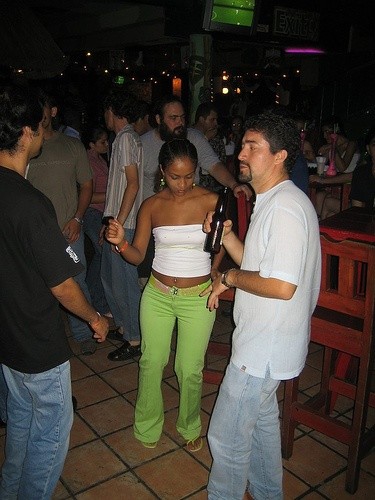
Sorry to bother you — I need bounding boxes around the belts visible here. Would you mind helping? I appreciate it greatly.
[150,273,211,296]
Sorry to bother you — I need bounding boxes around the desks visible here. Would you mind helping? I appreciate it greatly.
[309,173,353,212]
[319,205,374,414]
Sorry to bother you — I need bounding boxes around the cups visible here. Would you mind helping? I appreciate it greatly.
[316,151,327,174]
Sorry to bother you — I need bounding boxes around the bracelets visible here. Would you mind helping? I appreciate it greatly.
[72,216,80,222]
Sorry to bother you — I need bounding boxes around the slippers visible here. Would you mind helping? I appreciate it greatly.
[186,437,203,452]
[143,442,157,449]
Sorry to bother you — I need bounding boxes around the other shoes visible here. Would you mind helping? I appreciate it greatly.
[80,340,96,355]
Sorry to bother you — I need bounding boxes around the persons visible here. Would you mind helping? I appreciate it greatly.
[294,116,375,223]
[0,75,109,500]
[105,140,233,451]
[198,112,328,500]
[24,83,244,362]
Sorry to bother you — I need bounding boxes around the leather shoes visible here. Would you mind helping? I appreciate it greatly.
[107,327,129,343]
[108,342,140,362]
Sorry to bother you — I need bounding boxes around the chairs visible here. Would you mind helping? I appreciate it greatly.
[282,233,375,494]
[202,288,236,384]
[237,191,253,241]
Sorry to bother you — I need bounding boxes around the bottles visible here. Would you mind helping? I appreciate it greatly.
[204,186,230,254]
[327,133,338,177]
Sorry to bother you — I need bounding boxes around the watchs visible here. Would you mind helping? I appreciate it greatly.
[115,239,128,252]
[221,270,231,288]
